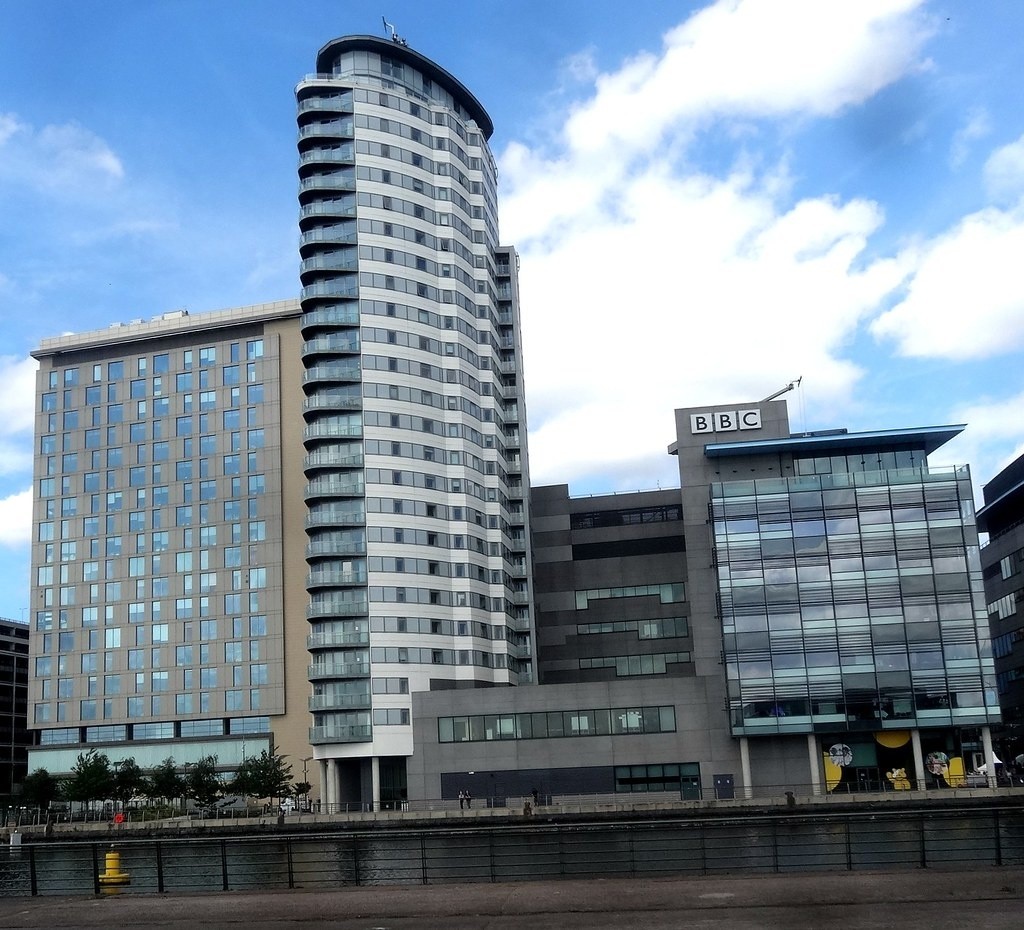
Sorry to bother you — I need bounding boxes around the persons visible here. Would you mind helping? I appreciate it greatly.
[316,796,321,812]
[459,791,464,809]
[530,785,540,807]
[464,790,472,809]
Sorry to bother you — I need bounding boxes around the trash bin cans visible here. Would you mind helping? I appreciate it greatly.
[680,776,702,800]
